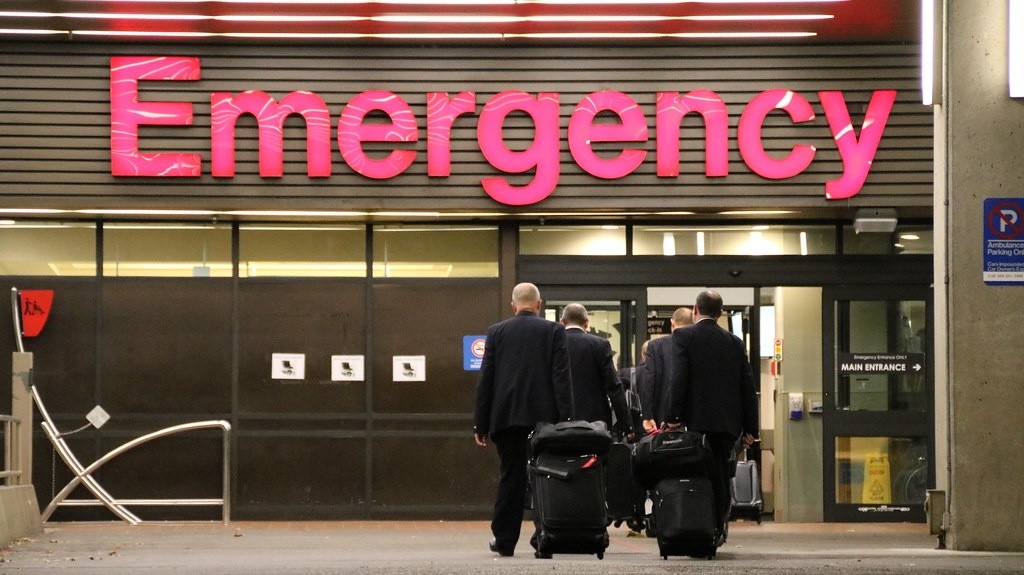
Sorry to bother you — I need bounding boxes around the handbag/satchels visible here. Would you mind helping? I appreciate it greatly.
[612,367,641,428]
[528,420,612,455]
[631,425,735,480]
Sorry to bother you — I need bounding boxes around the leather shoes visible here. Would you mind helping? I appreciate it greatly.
[489,540,514,557]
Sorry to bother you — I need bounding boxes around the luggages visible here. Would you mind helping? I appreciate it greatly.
[603,439,640,528]
[656,479,716,560]
[730,446,763,525]
[534,455,609,560]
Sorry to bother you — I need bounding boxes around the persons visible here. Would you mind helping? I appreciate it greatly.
[556,304,636,459]
[636,306,694,439]
[472,281,576,560]
[614,338,661,537]
[659,287,762,548]
